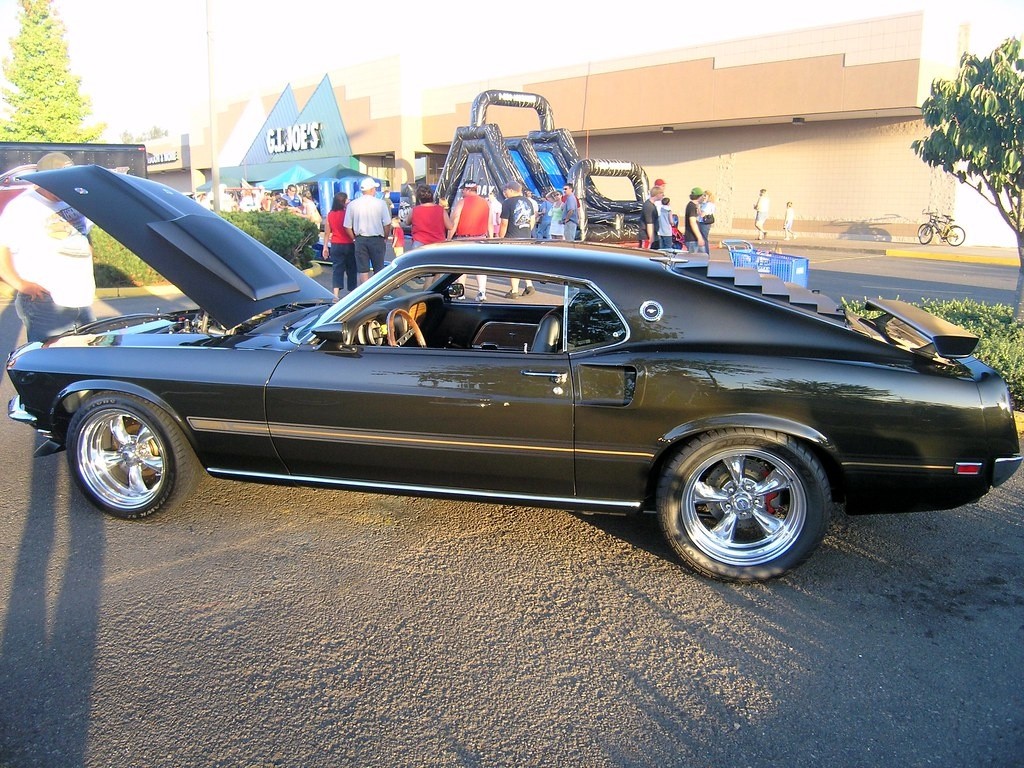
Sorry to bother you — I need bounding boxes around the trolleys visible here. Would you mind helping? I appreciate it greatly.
[722,240,809,289]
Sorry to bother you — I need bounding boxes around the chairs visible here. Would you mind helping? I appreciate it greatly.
[531,305,564,353]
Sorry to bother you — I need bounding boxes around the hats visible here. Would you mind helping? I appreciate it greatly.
[655,179,666,185]
[690,187,705,196]
[505,181,520,192]
[458,180,477,191]
[360,178,380,192]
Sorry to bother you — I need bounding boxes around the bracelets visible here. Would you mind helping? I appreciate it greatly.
[324,244,328,246]
[353,238,355,242]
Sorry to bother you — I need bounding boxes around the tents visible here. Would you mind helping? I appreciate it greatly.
[299,162,380,194]
[196,180,213,192]
[256,166,315,193]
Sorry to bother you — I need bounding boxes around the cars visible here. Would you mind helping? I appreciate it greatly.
[6,164,1023,585]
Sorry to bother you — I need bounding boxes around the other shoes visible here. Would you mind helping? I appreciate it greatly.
[784,237,789,240]
[763,232,768,240]
[755,235,762,240]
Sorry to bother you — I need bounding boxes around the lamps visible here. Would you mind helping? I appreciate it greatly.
[792,117,804,125]
[181,169,186,172]
[662,127,674,133]
[385,154,393,158]
[161,171,165,174]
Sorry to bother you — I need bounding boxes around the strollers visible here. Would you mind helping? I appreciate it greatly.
[672,226,689,251]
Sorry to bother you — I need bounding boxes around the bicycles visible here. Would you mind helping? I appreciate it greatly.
[918,209,966,246]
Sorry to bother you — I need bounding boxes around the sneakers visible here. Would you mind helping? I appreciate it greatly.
[521,286,536,296]
[452,295,467,300]
[504,290,520,300]
[475,290,487,302]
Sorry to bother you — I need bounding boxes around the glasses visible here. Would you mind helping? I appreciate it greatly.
[554,194,560,197]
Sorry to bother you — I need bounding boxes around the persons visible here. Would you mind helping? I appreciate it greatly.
[754,189,768,239]
[187,184,322,233]
[391,217,405,257]
[322,192,357,296]
[405,185,453,291]
[546,190,564,239]
[640,179,674,248]
[447,180,494,301]
[384,190,394,215]
[784,202,798,240]
[485,193,502,237]
[538,194,553,239]
[0,152,96,342]
[523,188,539,238]
[685,188,714,253]
[499,181,535,299]
[562,183,578,242]
[343,178,391,283]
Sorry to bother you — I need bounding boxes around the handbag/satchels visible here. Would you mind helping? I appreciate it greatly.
[547,208,554,217]
[703,214,715,224]
[535,215,543,223]
